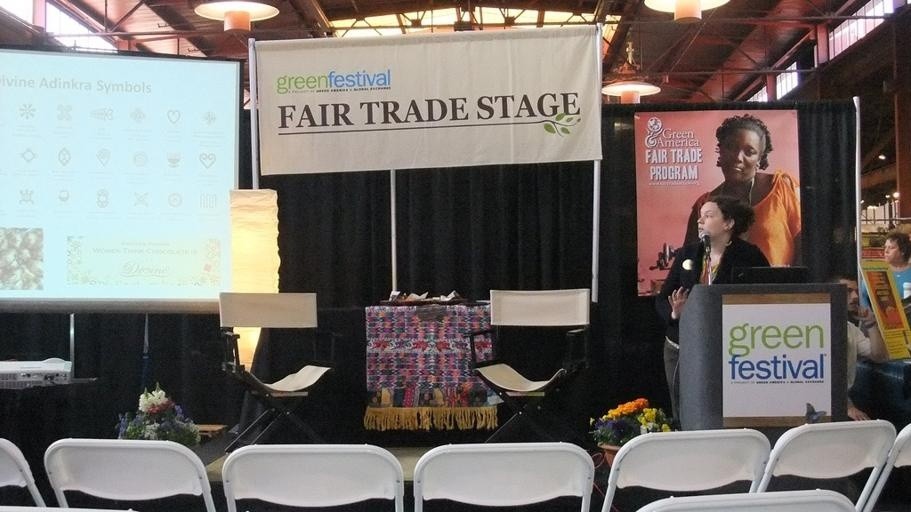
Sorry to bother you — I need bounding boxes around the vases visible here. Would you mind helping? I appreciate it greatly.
[597,442,622,468]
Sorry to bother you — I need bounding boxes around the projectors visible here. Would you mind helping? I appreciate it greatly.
[0,357,72,390]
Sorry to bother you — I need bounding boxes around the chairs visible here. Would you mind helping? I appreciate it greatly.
[222,444,405,512]
[43,438,216,512]
[1,436,46,507]
[635,489,855,512]
[863,423,911,511]
[757,420,897,512]
[413,442,595,512]
[602,429,771,512]
[462,288,590,443]
[220,331,331,452]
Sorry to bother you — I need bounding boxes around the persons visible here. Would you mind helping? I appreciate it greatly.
[823,271,890,421]
[861,233,911,317]
[656,195,770,424]
[682,115,802,267]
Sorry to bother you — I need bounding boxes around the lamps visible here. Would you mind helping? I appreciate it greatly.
[602,42,662,105]
[194,0,280,35]
[643,0,729,24]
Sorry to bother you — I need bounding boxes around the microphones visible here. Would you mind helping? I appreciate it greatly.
[699,231,713,256]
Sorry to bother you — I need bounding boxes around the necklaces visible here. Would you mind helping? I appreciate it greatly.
[717,178,754,207]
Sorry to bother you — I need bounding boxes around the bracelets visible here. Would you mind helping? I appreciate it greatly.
[863,319,876,328]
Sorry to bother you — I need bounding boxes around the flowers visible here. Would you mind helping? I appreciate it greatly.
[114,381,202,447]
[589,397,672,446]
